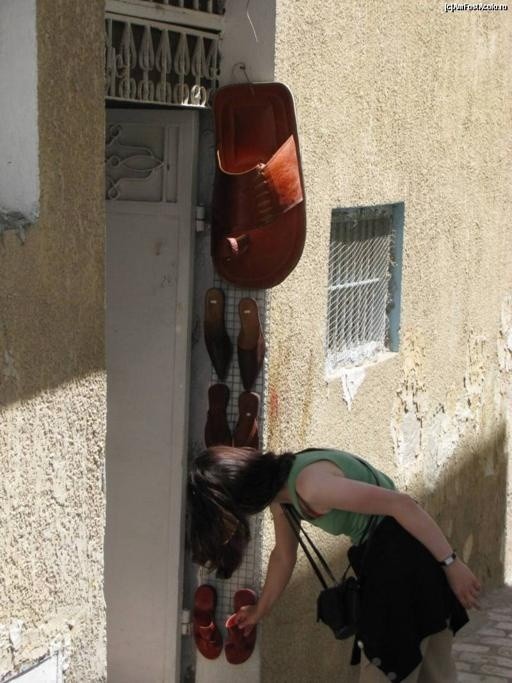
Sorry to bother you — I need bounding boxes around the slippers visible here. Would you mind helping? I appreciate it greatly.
[231,392,261,452]
[222,588,260,664]
[210,80,308,290]
[204,383,232,449]
[193,584,223,661]
[237,297,267,390]
[203,288,232,381]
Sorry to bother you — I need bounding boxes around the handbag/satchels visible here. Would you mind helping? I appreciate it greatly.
[315,573,366,640]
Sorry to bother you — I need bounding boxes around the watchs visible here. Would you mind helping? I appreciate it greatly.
[439,549,456,566]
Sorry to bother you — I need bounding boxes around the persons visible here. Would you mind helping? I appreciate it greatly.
[187,445,482,683]
